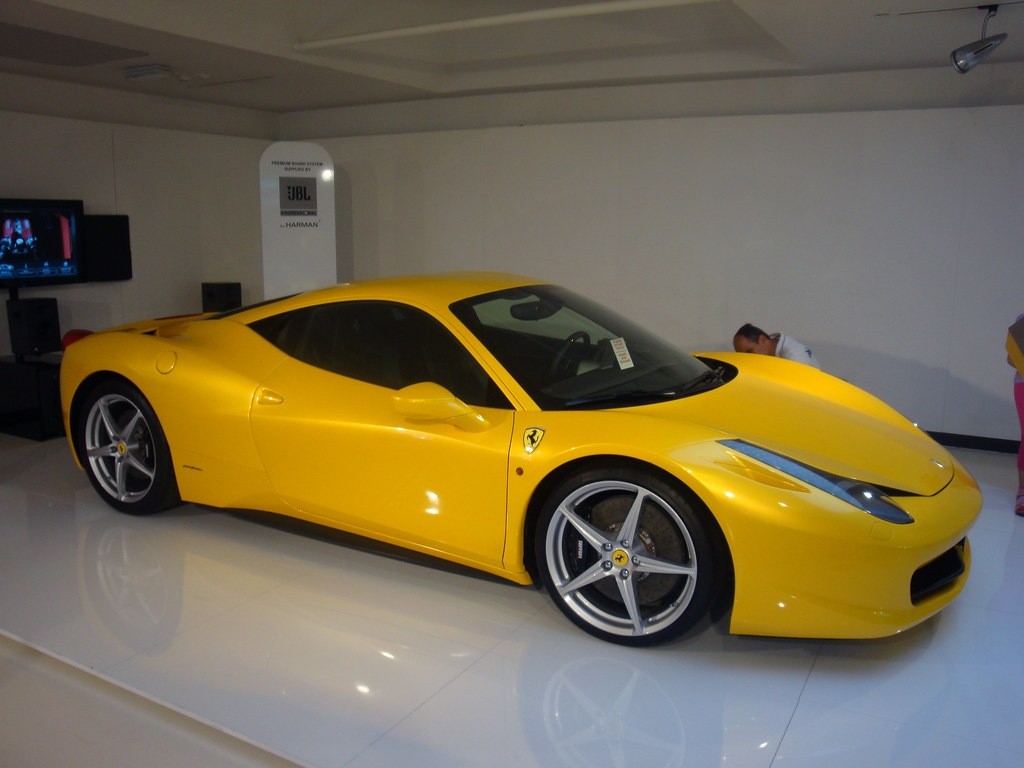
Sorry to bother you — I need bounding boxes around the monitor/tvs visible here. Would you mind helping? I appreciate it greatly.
[70,215,133,284]
[0,197,86,287]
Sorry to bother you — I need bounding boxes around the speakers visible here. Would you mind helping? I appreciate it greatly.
[201,281,242,313]
[7,298,62,362]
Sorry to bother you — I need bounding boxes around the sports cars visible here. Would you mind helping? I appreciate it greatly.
[58,271,987,649]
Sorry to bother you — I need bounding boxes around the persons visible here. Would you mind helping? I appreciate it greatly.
[733,323,820,370]
[1006,314,1024,515]
